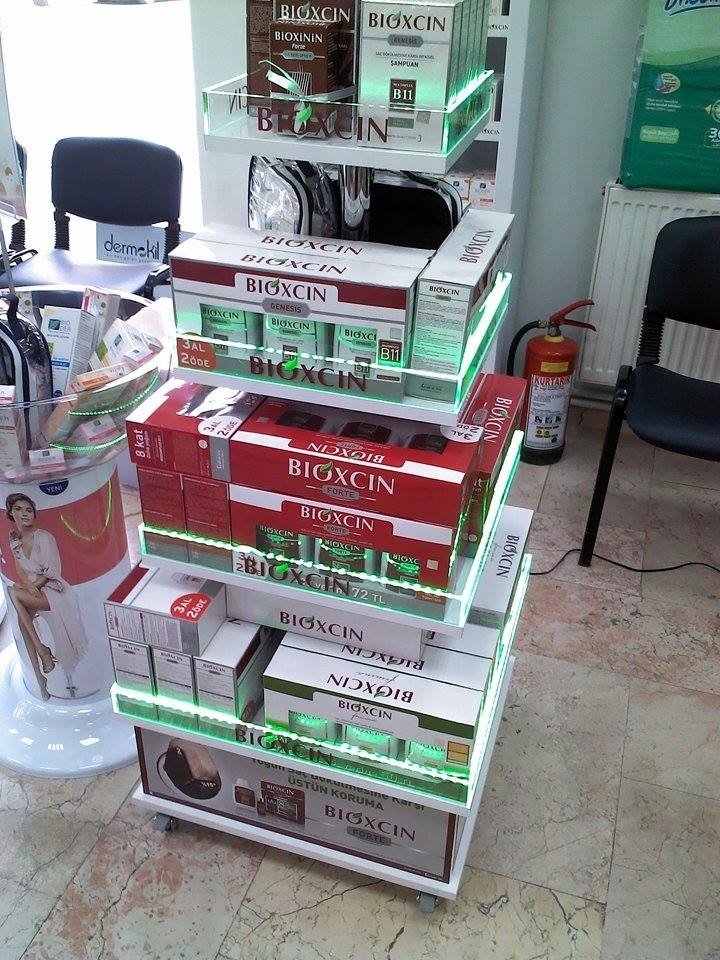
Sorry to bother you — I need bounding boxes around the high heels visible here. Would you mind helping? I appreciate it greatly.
[38,676,52,702]
[37,645,58,674]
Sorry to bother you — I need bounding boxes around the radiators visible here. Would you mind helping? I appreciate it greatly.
[575,179,720,398]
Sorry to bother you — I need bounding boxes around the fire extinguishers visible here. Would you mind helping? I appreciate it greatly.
[506,300,596,466]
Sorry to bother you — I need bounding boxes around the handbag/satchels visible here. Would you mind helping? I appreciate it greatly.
[326,158,462,249]
[245,153,338,240]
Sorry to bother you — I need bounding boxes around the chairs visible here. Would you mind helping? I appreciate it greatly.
[0,135,185,338]
[576,215,720,579]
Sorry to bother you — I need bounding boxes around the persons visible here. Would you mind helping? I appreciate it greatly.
[3,493,89,703]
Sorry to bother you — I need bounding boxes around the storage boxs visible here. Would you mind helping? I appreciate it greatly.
[0,284,167,485]
[103,0,538,888]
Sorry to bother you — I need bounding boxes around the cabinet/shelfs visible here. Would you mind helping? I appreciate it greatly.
[85,2,551,913]
